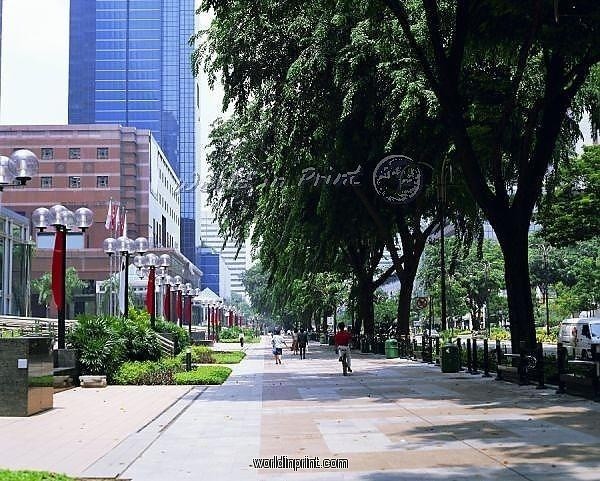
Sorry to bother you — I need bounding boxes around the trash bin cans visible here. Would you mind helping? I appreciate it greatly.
[320,335,326,344]
[329,334,335,346]
[384,338,399,359]
[440,344,460,373]
[360,338,369,353]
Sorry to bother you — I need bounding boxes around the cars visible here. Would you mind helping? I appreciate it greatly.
[423,329,439,341]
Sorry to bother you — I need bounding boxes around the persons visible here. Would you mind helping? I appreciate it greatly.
[571,327,579,347]
[335,321,353,372]
[238,330,245,347]
[259,326,351,364]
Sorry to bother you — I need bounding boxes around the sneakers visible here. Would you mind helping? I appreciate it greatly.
[348,367,352,373]
[276,359,281,364]
[339,356,342,362]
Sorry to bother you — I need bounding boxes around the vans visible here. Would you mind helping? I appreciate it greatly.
[556,317,600,362]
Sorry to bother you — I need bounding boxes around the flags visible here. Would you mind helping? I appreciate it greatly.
[104,200,127,239]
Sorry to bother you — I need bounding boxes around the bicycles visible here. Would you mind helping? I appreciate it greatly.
[339,349,351,376]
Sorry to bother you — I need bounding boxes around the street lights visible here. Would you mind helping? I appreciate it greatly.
[31,203,94,350]
[103,234,236,345]
